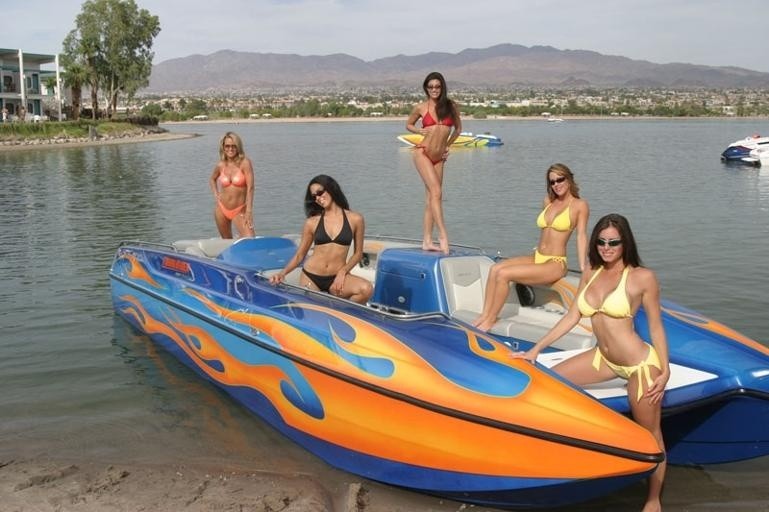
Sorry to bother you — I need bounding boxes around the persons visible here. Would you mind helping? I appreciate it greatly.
[1,107,10,123]
[470,164,589,333]
[19,105,26,122]
[507,212,673,512]
[404,70,462,256]
[267,174,375,306]
[208,131,257,239]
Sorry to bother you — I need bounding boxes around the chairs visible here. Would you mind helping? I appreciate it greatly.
[442,253,595,350]
[258,267,303,288]
[172,240,233,259]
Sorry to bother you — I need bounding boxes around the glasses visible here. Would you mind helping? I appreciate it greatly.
[427,85,441,89]
[550,176,565,185]
[597,237,622,246]
[311,188,326,201]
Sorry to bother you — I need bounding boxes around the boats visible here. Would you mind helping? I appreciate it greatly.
[109,236,769,511]
[396,131,504,149]
[547,118,564,123]
[721,135,769,162]
[740,144,769,165]
